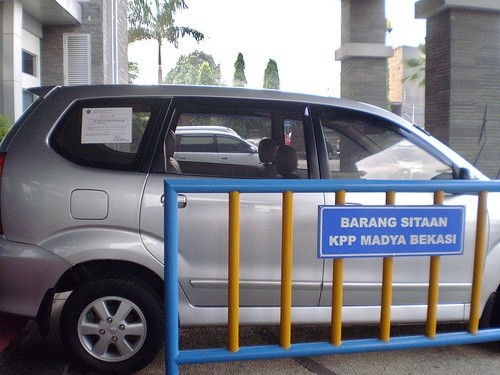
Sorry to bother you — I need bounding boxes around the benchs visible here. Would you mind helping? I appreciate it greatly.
[168,129,189,174]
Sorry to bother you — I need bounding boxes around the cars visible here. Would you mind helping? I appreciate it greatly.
[0,84,499,375]
[166,129,267,167]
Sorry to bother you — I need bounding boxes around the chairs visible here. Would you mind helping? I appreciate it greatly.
[256,138,277,179]
[275,145,307,181]
